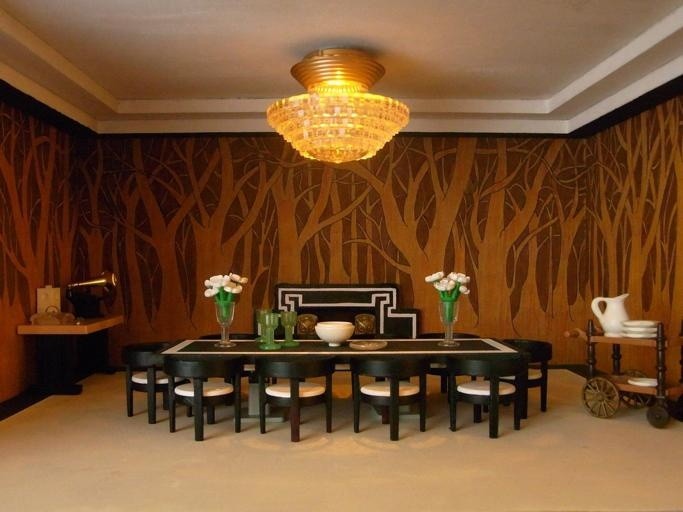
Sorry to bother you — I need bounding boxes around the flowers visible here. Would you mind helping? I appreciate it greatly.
[424,270,471,322]
[204,272,248,321]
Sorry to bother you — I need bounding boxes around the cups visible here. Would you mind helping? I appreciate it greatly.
[257,313,280,349]
[255,309,269,342]
[279,312,299,347]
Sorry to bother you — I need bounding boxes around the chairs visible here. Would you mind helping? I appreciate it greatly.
[199,333,258,406]
[351,356,427,441]
[418,333,481,393]
[122,343,191,424]
[447,356,521,438]
[499,339,552,419]
[164,355,241,441]
[272,334,305,385]
[255,357,335,442]
[350,334,388,381]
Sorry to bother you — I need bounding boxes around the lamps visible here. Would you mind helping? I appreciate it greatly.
[266,48,410,164]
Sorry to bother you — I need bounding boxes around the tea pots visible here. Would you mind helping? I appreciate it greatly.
[591,294,629,338]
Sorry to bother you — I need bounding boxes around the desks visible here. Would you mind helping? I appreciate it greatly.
[16,312,125,395]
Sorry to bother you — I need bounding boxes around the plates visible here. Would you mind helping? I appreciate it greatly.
[628,378,657,387]
[349,341,387,350]
[622,320,659,340]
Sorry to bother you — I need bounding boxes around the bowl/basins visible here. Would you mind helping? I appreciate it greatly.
[314,321,354,347]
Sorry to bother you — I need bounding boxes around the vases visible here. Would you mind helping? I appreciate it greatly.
[214,301,237,348]
[437,301,460,347]
